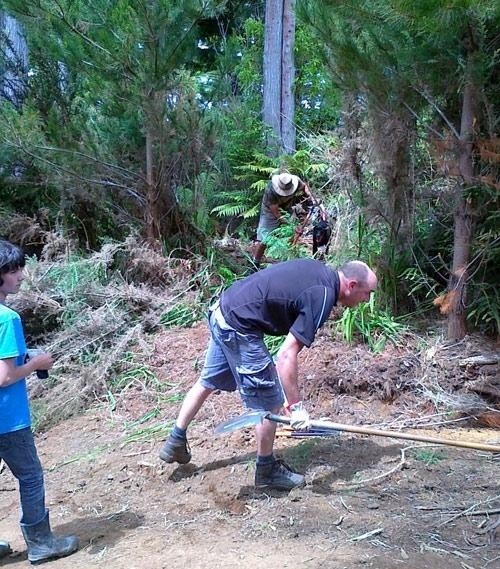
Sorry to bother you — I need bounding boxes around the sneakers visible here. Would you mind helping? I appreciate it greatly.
[159,439,192,465]
[255,460,304,491]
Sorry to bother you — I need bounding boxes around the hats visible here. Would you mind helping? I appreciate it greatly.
[271,171,299,196]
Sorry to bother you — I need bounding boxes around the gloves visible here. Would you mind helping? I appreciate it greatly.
[288,401,311,432]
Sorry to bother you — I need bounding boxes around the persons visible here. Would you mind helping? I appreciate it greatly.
[1,239,82,566]
[252,170,316,270]
[156,258,379,489]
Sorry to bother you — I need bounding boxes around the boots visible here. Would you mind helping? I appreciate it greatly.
[0,540,11,558]
[18,509,80,563]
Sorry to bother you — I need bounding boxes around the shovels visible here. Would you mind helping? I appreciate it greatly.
[214,411,500,452]
